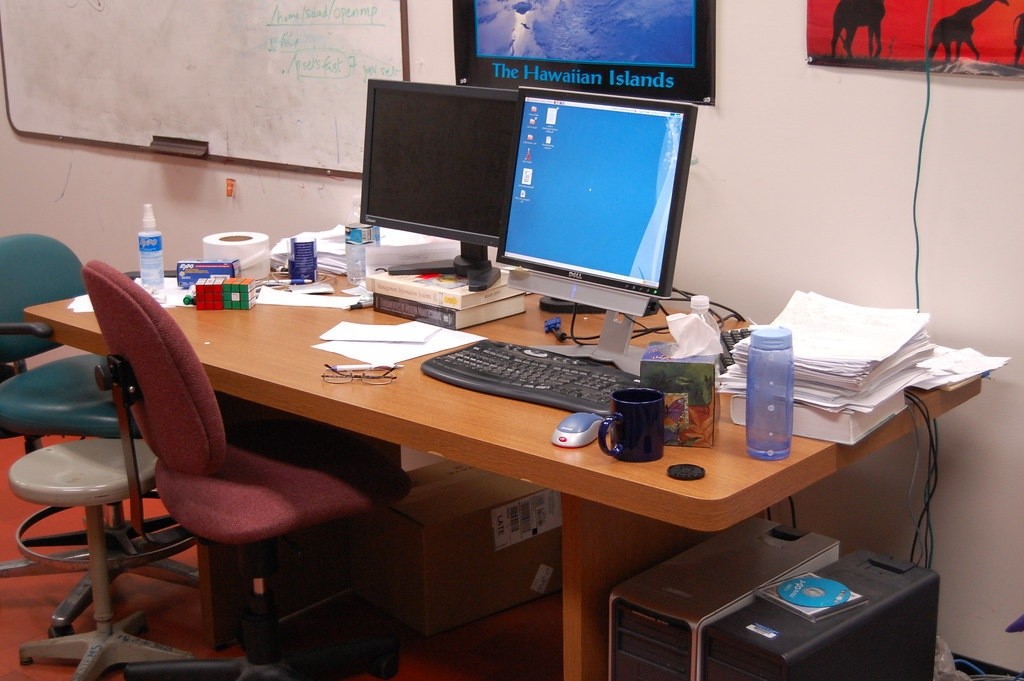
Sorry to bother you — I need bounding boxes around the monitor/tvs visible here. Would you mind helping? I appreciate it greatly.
[492,85,699,377]
[358,78,518,291]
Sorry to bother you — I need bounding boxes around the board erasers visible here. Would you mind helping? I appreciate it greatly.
[149,135,209,157]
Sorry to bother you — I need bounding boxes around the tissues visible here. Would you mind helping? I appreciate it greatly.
[639,311,725,449]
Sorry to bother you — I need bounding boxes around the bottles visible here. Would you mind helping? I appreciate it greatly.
[138,204,165,289]
[747,327,794,462]
[344,195,381,287]
[689,294,722,425]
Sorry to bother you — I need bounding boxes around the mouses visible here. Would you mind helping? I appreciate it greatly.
[551,412,609,448]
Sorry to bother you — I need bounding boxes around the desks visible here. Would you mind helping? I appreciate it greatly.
[23,253,982,681]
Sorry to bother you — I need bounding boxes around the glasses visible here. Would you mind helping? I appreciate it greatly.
[321,364,399,384]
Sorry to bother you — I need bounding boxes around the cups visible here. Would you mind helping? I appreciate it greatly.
[598,387,665,462]
[286,236,318,282]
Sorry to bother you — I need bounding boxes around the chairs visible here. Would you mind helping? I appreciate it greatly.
[1,233,150,449]
[79,260,401,681]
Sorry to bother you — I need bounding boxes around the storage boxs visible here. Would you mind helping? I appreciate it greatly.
[370,457,563,635]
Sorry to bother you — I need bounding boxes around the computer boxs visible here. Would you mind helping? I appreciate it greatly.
[608,516,839,681]
[703,548,940,681]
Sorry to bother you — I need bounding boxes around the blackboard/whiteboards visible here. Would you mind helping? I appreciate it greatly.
[0,0,410,178]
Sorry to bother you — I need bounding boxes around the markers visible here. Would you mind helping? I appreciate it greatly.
[183,285,196,305]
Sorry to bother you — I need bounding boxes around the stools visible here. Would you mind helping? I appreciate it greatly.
[9,439,196,681]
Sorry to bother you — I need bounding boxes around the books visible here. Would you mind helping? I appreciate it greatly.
[730,386,908,445]
[365,272,527,331]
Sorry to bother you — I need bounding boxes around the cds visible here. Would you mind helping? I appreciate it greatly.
[777,577,851,607]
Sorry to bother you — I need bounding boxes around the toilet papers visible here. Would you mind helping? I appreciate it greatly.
[203,230,272,280]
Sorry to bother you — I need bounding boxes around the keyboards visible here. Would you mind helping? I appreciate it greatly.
[420,339,641,418]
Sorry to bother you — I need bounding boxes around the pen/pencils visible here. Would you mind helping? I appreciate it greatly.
[327,364,405,371]
[350,301,373,310]
[263,279,313,285]
